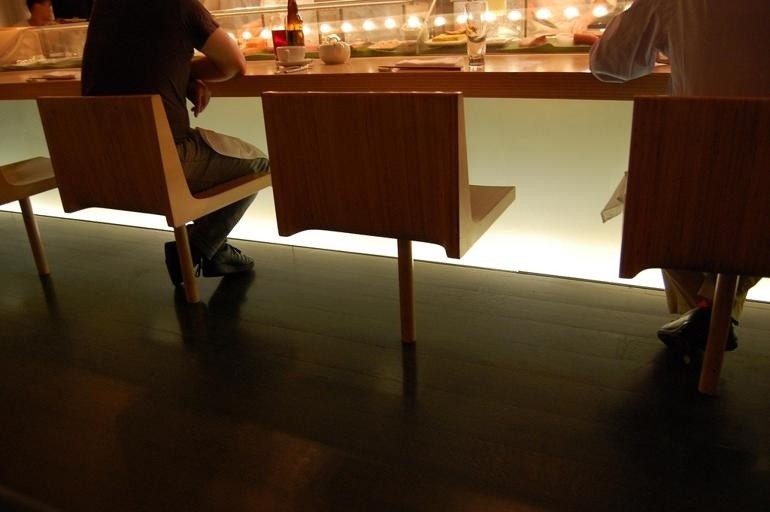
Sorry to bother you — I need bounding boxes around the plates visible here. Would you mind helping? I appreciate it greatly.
[368,40,402,51]
[277,58,314,68]
[378,55,463,70]
[424,30,592,49]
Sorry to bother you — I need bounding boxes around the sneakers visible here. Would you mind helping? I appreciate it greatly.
[202,244,255,277]
[164,241,185,287]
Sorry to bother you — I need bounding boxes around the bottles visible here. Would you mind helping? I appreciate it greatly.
[286,0,304,46]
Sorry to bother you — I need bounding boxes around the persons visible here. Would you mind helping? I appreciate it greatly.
[80,0,274,293]
[583,1,768,353]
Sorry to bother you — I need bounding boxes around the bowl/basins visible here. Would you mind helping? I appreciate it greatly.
[319,44,351,64]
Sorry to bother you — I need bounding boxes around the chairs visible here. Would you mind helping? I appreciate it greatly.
[618,94,770,396]
[36,92,272,328]
[260,90,516,347]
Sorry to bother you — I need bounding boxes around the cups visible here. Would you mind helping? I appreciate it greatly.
[276,45,307,63]
[463,1,486,67]
[270,16,288,56]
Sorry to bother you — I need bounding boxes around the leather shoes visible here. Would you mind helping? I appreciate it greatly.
[724,325,737,352]
[657,307,710,349]
